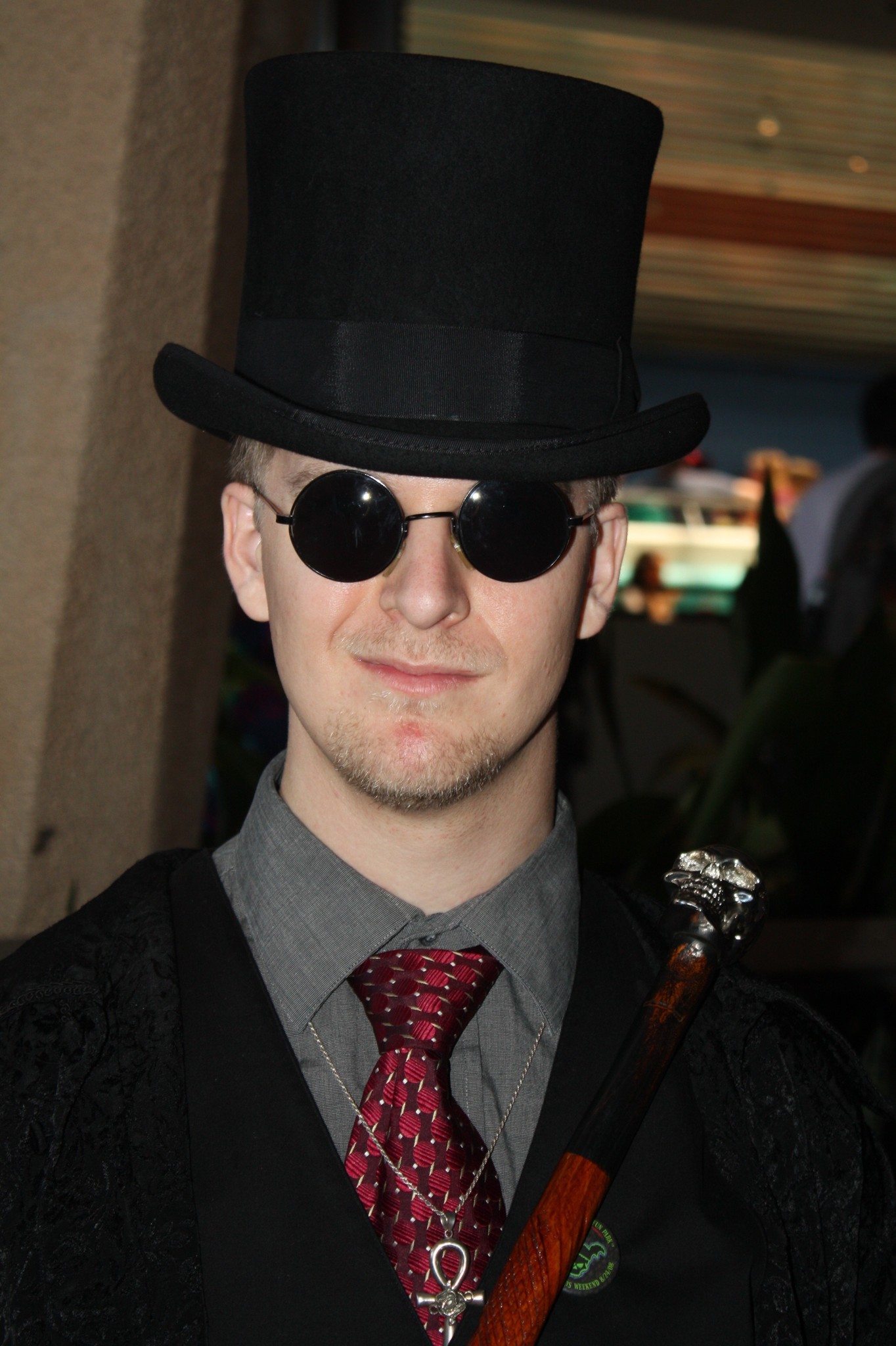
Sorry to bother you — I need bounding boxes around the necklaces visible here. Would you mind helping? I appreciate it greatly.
[307,1022,545,1346]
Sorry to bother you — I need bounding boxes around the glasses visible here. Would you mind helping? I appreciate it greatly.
[241,472,617,584]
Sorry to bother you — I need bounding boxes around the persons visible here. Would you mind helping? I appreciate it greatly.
[0,47,896,1346]
[786,363,896,611]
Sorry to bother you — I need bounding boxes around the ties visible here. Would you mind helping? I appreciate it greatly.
[341,943,511,1346]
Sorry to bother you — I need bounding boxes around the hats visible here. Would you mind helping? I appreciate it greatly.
[156,43,716,482]
